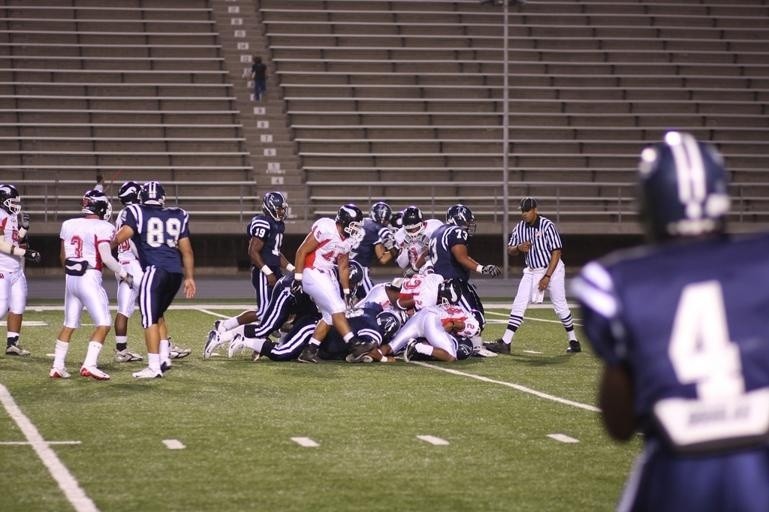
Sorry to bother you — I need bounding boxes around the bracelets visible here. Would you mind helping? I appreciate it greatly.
[545,274,551,277]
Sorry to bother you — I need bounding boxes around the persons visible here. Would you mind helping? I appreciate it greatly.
[201,189,504,365]
[113,180,192,363]
[484,198,583,354]
[49,190,134,380]
[0,182,42,356]
[94,176,110,193]
[110,180,197,379]
[570,130,769,511]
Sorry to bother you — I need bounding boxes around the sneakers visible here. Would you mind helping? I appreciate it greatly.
[403,337,420,366]
[111,348,146,363]
[47,366,74,380]
[78,362,112,381]
[202,330,220,362]
[564,338,583,355]
[3,343,32,359]
[344,338,397,364]
[166,335,194,361]
[215,320,228,350]
[228,332,246,360]
[250,334,272,363]
[484,338,513,357]
[297,345,327,365]
[131,353,173,381]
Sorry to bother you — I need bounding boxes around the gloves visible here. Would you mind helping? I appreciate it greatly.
[402,264,420,278]
[344,289,353,309]
[22,248,42,265]
[401,234,413,250]
[476,262,503,281]
[417,232,431,248]
[123,271,134,291]
[21,212,32,230]
[290,277,304,297]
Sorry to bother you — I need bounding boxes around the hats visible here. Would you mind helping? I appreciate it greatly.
[516,195,538,212]
[96,174,103,183]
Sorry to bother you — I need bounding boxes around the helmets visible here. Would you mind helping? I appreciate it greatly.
[140,180,167,206]
[468,307,486,332]
[261,189,289,224]
[635,128,735,239]
[0,183,22,215]
[445,203,476,227]
[335,202,366,237]
[438,277,463,304]
[455,336,474,360]
[370,201,425,231]
[347,261,364,286]
[375,311,400,343]
[117,180,142,199]
[80,189,113,222]
